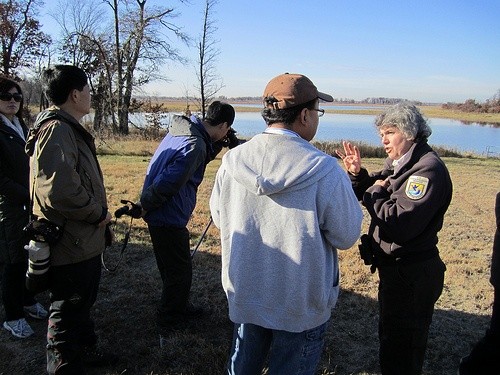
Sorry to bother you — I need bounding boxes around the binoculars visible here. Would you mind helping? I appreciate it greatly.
[114,205,142,219]
[358,234,379,266]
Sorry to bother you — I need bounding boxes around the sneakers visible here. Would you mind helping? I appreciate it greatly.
[23,301,48,320]
[3,318,34,338]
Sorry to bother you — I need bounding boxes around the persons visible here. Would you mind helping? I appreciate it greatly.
[91,86,105,131]
[0,77,48,338]
[209,73,363,375]
[459,191,500,375]
[140,101,235,326]
[334,103,453,375]
[226,126,239,148]
[27,66,119,375]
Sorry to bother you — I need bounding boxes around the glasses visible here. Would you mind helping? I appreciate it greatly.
[0,92,23,103]
[310,107,325,118]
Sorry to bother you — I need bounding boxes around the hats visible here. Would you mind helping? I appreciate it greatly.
[264,73,334,110]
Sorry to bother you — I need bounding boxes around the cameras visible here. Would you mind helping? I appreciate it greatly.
[23,218,62,294]
[217,130,248,150]
